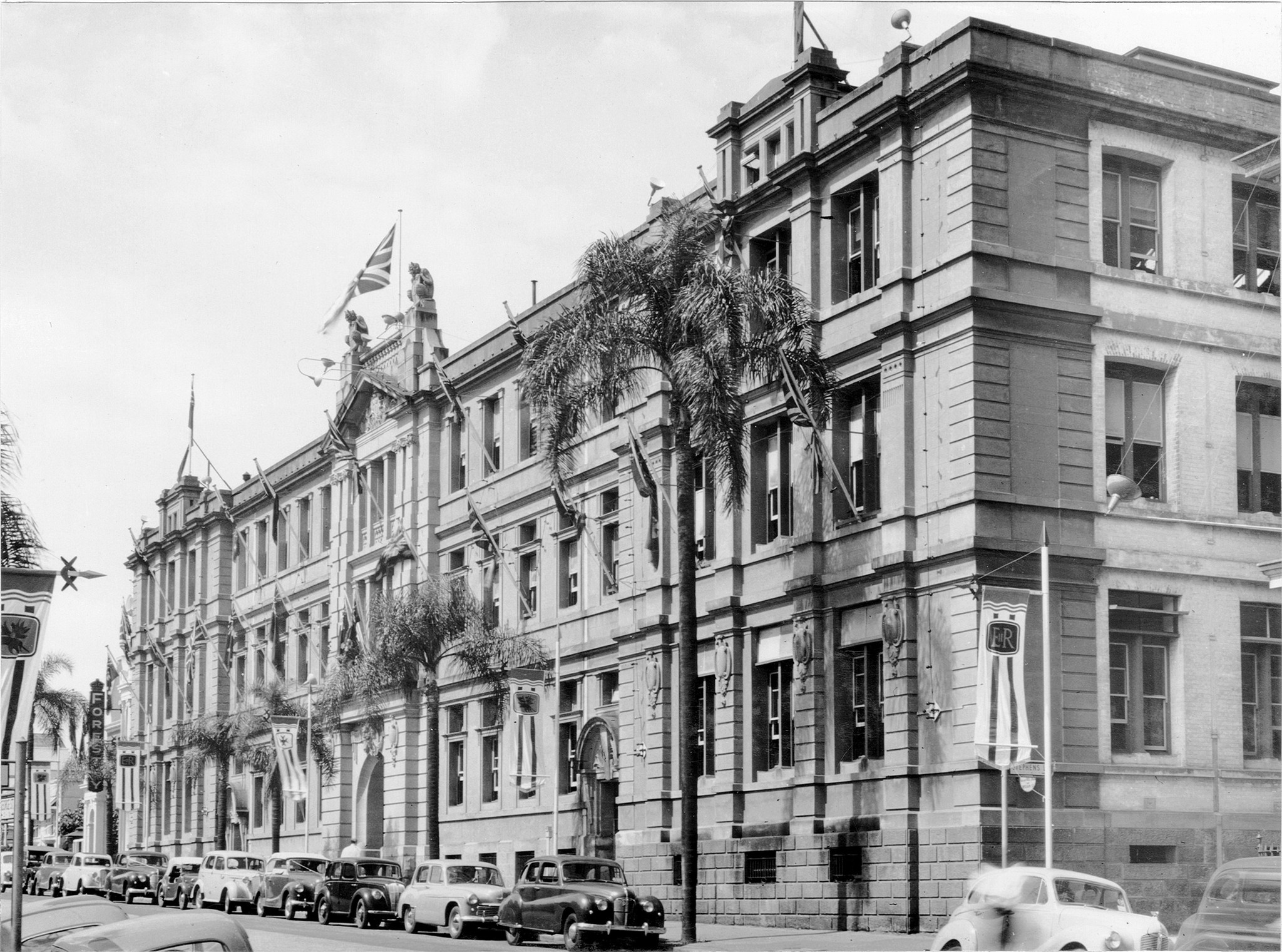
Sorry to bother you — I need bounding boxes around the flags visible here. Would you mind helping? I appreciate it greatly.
[434,357,461,424]
[216,492,240,563]
[345,591,362,665]
[551,468,586,540]
[627,424,657,497]
[505,306,530,351]
[118,601,136,672]
[703,179,736,255]
[185,613,209,688]
[222,599,248,677]
[317,222,396,337]
[373,523,418,585]
[268,582,290,684]
[337,599,350,665]
[106,652,120,716]
[256,462,280,545]
[466,498,497,554]
[327,416,362,503]
[777,349,816,427]
[146,630,171,700]
[132,533,154,579]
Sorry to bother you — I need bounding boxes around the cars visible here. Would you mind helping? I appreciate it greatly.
[50,908,253,952]
[492,855,667,952]
[251,852,334,920]
[928,862,1172,951]
[190,850,268,914]
[99,849,170,905]
[1169,855,1281,951]
[0,844,115,898]
[154,856,214,910]
[0,892,130,952]
[312,857,409,929]
[394,858,513,940]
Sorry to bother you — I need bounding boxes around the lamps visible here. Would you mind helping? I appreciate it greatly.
[1106,473,1142,515]
